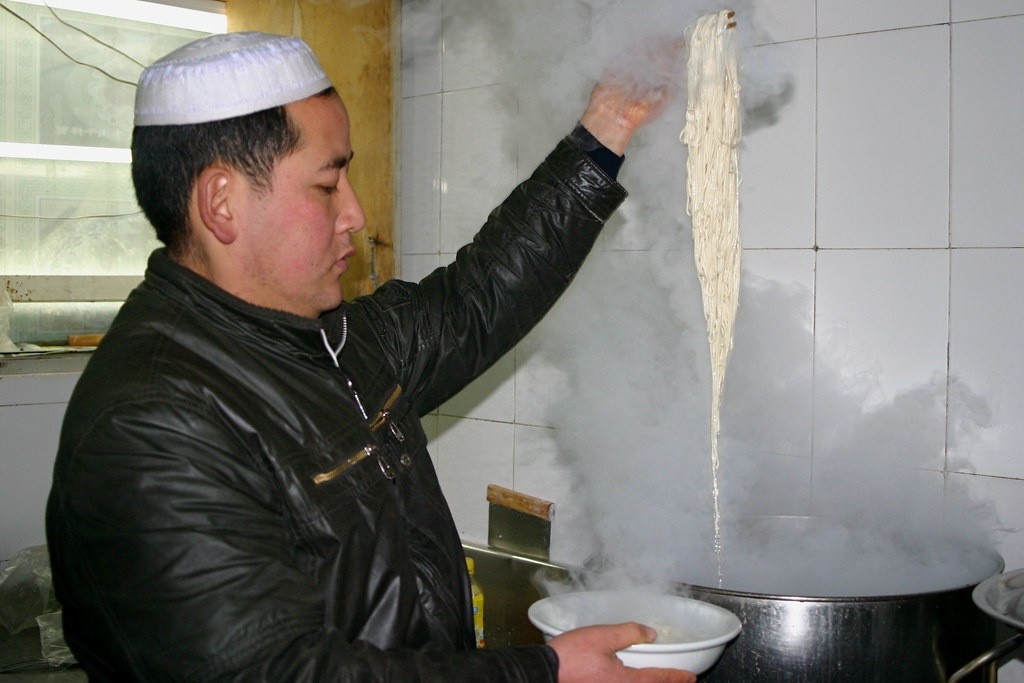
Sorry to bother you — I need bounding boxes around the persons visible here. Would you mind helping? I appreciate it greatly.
[42,29,700,683]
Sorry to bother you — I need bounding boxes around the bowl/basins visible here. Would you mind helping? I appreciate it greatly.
[526,590,743,678]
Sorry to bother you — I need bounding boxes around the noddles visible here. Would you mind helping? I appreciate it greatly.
[678,9,746,544]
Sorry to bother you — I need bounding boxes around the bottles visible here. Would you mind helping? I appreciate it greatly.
[465,557,484,650]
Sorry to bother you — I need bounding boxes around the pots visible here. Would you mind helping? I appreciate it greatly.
[657,538,1005,683]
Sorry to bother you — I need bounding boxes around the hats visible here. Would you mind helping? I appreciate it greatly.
[130,29,335,133]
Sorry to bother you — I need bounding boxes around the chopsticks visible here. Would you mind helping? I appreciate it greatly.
[663,10,738,50]
